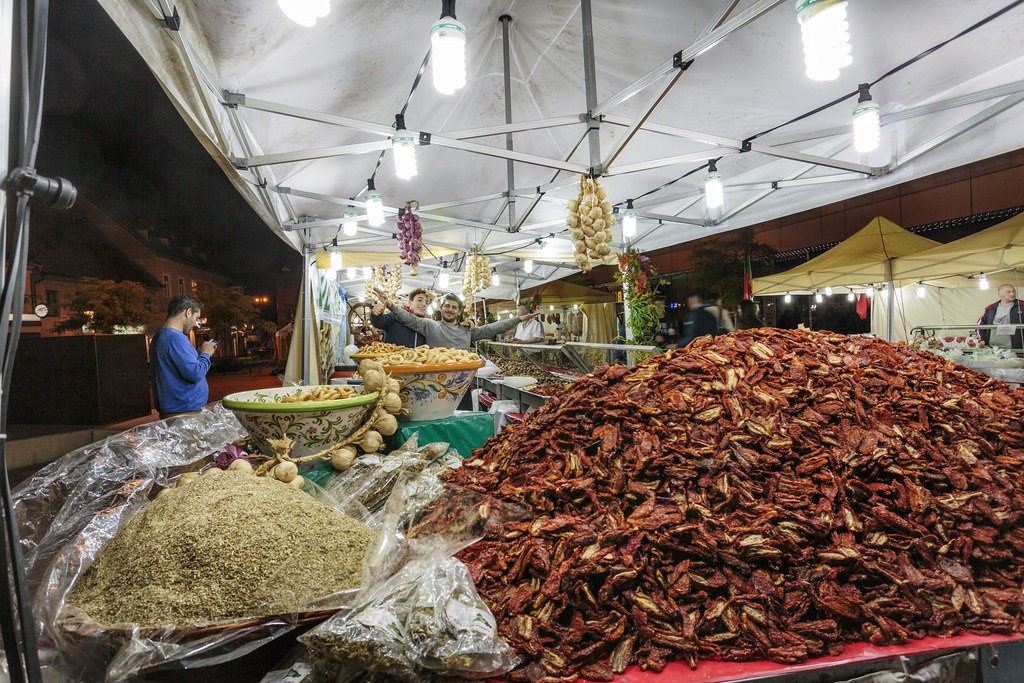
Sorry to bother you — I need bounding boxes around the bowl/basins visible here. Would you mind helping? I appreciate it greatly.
[384,360,485,421]
[223,384,381,462]
[350,354,395,365]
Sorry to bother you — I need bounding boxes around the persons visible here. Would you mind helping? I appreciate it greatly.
[654,296,680,353]
[510,305,546,355]
[666,292,717,350]
[975,283,1024,348]
[369,287,436,347]
[703,286,736,335]
[734,299,767,330]
[370,287,541,413]
[150,294,217,419]
[614,325,628,365]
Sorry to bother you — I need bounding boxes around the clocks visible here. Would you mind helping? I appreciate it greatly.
[34,303,48,318]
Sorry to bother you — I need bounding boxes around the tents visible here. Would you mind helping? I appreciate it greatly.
[884,209,1024,344]
[472,279,617,367]
[749,214,944,341]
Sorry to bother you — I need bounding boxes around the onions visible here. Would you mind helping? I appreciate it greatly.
[394,208,422,277]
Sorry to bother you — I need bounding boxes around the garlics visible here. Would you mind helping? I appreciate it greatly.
[566,187,614,273]
[362,265,400,302]
[462,257,490,313]
[157,359,400,503]
